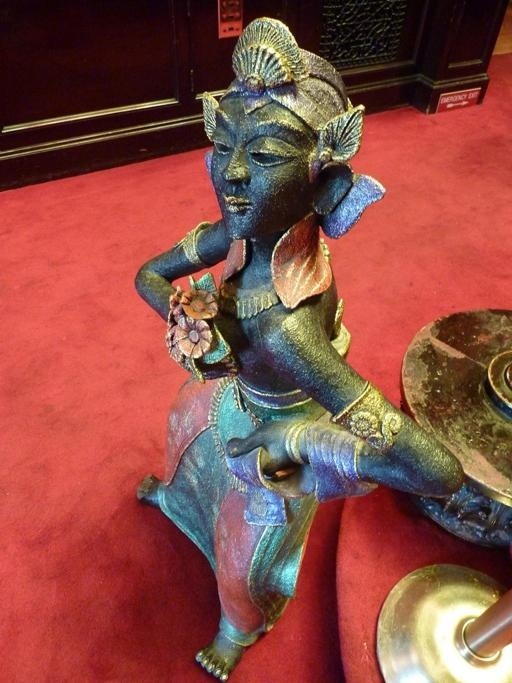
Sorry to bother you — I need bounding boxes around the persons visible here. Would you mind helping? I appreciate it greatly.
[133,16,465,683]
